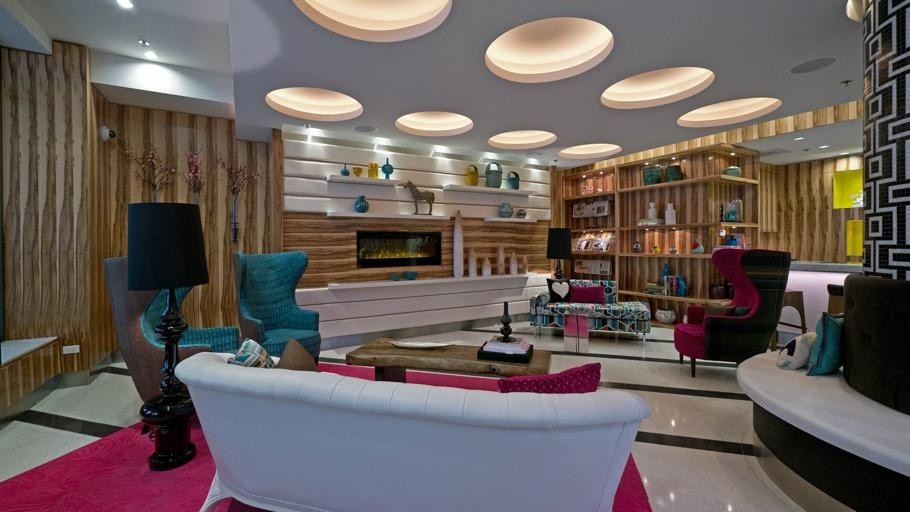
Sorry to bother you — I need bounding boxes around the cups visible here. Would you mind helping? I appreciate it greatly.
[668,275,678,296]
[368,163,379,179]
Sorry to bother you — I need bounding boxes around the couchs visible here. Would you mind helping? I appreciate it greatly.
[172,351,650,512]
[527,279,652,343]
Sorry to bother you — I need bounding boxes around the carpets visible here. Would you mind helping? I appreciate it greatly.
[2,361,655,512]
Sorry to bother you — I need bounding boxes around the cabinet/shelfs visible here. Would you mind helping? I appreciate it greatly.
[560,143,760,308]
[326,173,541,224]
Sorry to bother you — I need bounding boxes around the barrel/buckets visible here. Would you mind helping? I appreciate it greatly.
[664,165,682,182]
[643,164,662,185]
[464,165,478,186]
[506,171,520,190]
[485,161,503,187]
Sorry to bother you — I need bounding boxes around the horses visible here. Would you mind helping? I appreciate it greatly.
[403,180,436,214]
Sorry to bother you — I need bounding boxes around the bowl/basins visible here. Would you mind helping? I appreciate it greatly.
[353,167,363,178]
[391,274,400,281]
[405,271,416,279]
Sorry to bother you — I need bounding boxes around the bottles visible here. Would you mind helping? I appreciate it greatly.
[468,245,477,277]
[521,254,529,274]
[648,201,657,220]
[495,245,506,275]
[664,203,676,225]
[452,210,464,277]
[481,256,492,276]
[663,262,669,295]
[731,198,743,222]
[508,251,518,275]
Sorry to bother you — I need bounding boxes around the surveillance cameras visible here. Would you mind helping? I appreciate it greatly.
[101,128,116,142]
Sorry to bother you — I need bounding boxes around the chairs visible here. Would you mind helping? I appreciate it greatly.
[673,247,791,379]
[104,256,241,403]
[233,251,322,370]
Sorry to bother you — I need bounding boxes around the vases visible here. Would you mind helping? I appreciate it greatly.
[195,193,201,208]
[231,196,238,243]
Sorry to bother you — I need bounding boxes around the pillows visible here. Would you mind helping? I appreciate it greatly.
[735,306,753,317]
[805,311,846,377]
[226,337,276,369]
[545,277,571,303]
[774,332,816,370]
[496,361,601,393]
[570,285,606,304]
[702,299,737,316]
[276,337,319,370]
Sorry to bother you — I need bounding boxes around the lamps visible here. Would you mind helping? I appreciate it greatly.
[547,227,575,278]
[126,201,210,471]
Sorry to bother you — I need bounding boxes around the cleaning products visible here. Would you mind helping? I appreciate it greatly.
[676,274,686,296]
[648,202,676,225]
[659,264,671,285]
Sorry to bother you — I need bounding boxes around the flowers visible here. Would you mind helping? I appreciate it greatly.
[184,149,204,191]
[114,133,173,200]
[214,155,268,196]
